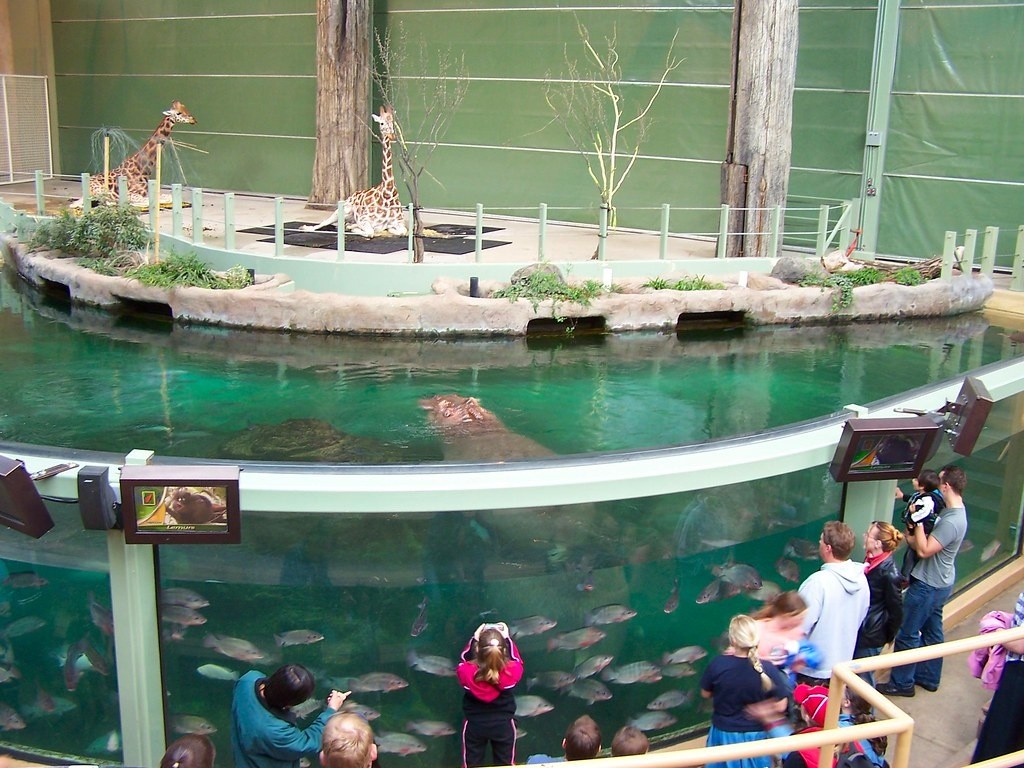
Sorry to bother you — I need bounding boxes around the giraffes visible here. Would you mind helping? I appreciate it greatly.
[297,106,407,239]
[89,101,197,201]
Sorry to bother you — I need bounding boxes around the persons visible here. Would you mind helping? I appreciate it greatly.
[319,710,377,768]
[796,518,869,689]
[230,664,352,768]
[159,733,216,768]
[853,521,904,715]
[972,592,1024,768]
[456,621,524,768]
[700,591,888,768]
[877,465,967,695]
[611,726,649,757]
[526,715,601,764]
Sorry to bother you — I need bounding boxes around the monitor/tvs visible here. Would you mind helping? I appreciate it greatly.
[829,416,940,483]
[0,455,54,539]
[946,376,995,456]
[118,465,241,545]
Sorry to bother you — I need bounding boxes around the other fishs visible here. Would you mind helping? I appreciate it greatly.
[0,537,1024,758]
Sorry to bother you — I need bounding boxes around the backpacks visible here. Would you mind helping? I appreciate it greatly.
[834,742,877,768]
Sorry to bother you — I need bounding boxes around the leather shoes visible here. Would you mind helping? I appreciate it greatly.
[875,683,915,697]
[914,682,937,692]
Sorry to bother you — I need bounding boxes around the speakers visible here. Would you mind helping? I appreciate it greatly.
[922,414,944,463]
[77,467,117,530]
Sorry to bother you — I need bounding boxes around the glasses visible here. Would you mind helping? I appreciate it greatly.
[863,533,877,540]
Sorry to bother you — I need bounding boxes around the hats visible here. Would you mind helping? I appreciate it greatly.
[794,684,829,727]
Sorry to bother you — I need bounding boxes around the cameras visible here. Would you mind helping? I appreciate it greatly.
[482,624,504,632]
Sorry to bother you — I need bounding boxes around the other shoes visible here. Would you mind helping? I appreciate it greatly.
[901,581,909,590]
